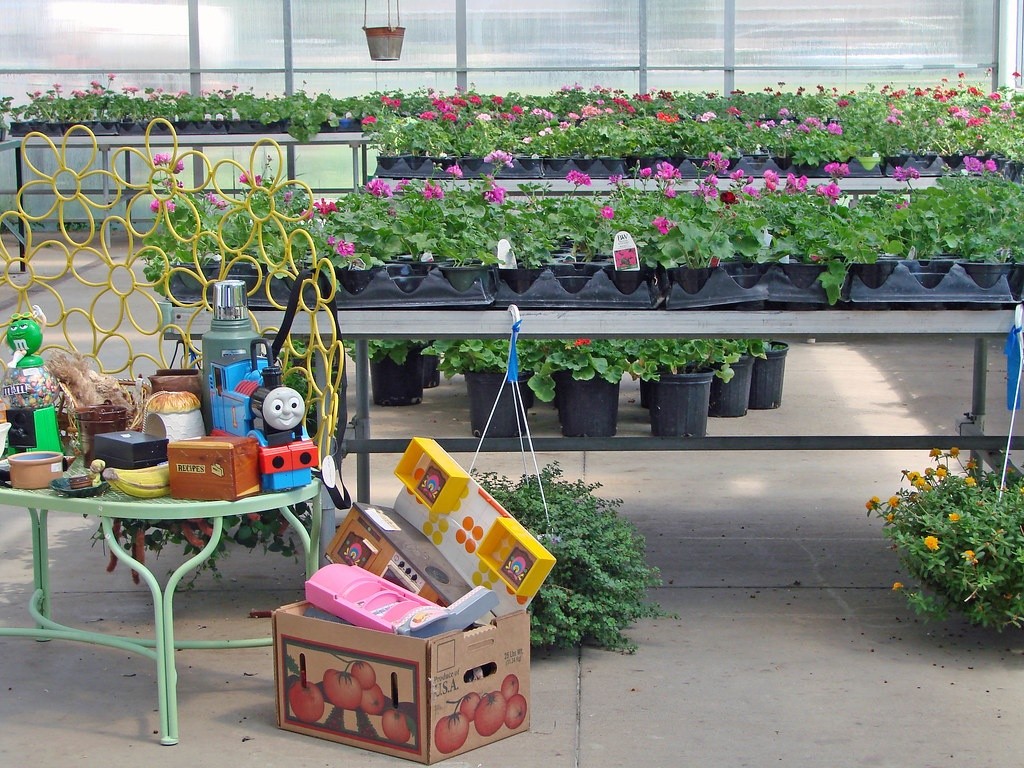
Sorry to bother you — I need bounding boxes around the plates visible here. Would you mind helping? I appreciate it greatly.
[49,477,109,497]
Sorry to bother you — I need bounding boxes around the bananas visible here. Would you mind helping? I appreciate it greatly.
[102,464,171,499]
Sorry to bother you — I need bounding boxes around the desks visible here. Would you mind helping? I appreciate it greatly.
[372,178,1021,193]
[0,479,323,743]
[157,305,1024,505]
[0,138,26,272]
[11,133,378,235]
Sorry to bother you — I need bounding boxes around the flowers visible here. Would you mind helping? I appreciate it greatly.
[0,73,1024,255]
[866,447,1024,634]
[573,339,590,348]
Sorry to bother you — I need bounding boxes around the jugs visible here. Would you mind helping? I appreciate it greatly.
[203,280,262,435]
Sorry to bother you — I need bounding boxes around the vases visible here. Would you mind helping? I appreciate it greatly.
[160,262,1021,310]
[727,158,739,170]
[572,158,591,172]
[379,156,401,170]
[970,155,985,164]
[601,158,626,172]
[744,154,769,169]
[518,158,542,171]
[900,154,919,169]
[410,155,430,170]
[991,155,1005,167]
[688,158,709,169]
[997,158,1010,171]
[947,155,963,168]
[460,157,484,172]
[365,27,404,61]
[928,156,946,168]
[1004,162,1022,180]
[368,343,790,440]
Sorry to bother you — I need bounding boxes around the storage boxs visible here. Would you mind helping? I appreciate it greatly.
[271,600,530,765]
[168,436,260,504]
[96,430,169,481]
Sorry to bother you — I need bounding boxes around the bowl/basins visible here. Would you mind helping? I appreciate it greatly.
[8,451,64,489]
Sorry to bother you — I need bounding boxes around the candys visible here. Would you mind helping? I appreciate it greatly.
[0,364,60,410]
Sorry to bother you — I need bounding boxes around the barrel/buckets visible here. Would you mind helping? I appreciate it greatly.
[74,400,134,468]
[366,27,406,60]
